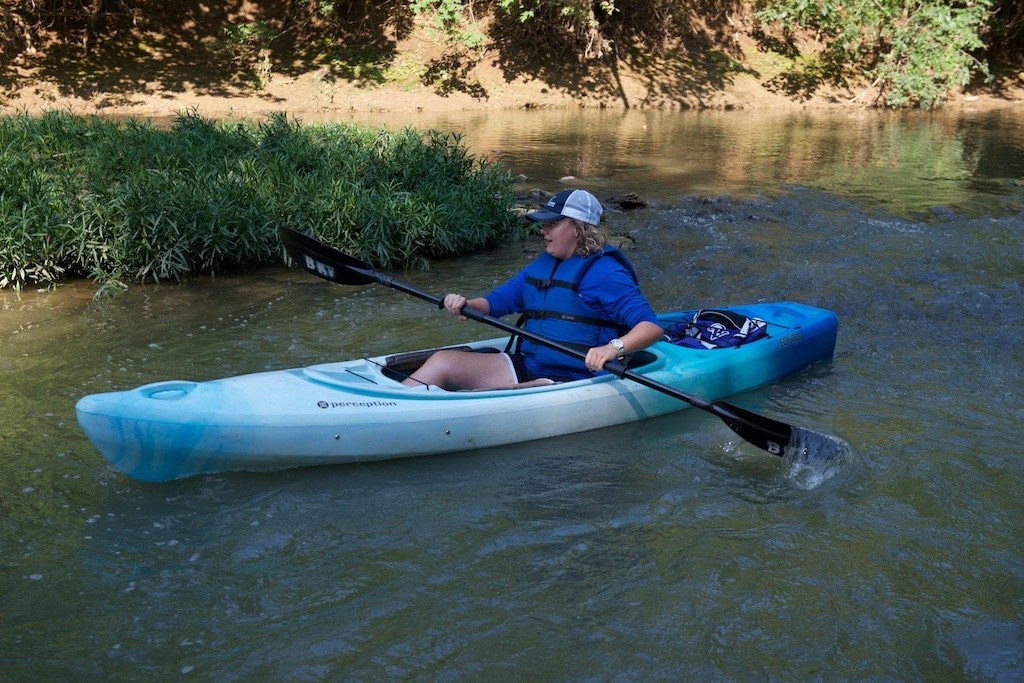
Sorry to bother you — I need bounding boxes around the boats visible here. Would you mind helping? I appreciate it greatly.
[76,299,840,480]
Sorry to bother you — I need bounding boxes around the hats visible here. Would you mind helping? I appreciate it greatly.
[525,188,604,227]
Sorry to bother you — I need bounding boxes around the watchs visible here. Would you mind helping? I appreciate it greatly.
[610,339,624,360]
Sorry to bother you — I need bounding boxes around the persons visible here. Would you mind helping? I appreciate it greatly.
[399,188,664,386]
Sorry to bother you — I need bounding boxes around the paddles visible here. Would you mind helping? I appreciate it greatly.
[278,228,791,456]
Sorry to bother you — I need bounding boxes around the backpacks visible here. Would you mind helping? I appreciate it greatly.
[658,307,768,350]
